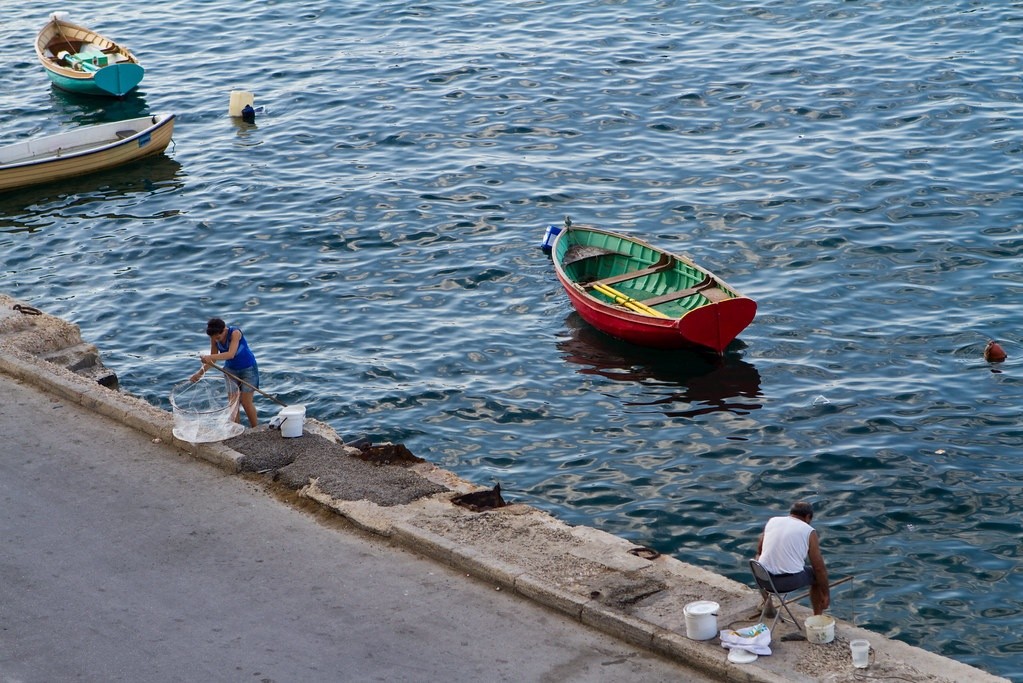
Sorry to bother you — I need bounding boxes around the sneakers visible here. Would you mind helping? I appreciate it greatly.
[757,601,776,618]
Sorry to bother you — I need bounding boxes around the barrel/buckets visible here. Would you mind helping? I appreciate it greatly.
[277,411,303,438]
[681,601,723,643]
[804,615,837,645]
[282,403,307,422]
[539,225,561,250]
[230,89,255,119]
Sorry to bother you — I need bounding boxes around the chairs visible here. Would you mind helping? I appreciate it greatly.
[749,559,802,635]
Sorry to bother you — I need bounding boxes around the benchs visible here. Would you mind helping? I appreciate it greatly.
[117,130,138,138]
[615,275,715,310]
[48,44,117,60]
[581,253,673,288]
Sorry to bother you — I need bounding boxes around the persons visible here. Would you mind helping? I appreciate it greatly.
[751,500,832,622]
[186,316,261,431]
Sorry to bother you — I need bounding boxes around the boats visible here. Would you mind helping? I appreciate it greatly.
[0,114,176,194]
[34,13,145,99]
[549,217,762,352]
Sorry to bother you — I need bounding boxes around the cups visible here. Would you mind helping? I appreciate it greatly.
[850,638,871,670]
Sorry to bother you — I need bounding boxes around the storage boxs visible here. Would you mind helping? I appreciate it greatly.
[75,53,92,64]
[85,47,109,67]
